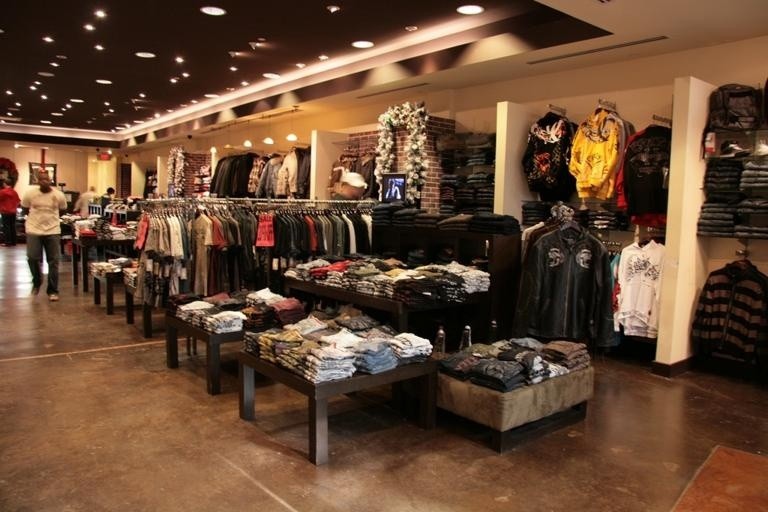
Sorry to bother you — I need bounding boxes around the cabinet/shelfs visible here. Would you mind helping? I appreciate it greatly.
[372,224,522,316]
[698,124,767,240]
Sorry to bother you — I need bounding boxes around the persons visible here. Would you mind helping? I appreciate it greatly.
[19,173,67,300]
[385,181,401,199]
[0,172,7,188]
[74,186,100,216]
[100,186,114,215]
[0,179,21,246]
[332,171,368,209]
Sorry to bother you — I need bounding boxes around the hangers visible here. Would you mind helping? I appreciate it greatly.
[540,96,673,136]
[262,143,311,167]
[142,196,376,221]
[602,225,664,262]
[338,143,376,166]
[731,250,753,268]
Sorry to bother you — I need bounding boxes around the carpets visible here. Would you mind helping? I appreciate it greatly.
[668,444,768,511]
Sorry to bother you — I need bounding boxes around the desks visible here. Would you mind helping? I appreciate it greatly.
[165,307,267,395]
[239,349,435,465]
[281,277,487,333]
[60,198,153,338]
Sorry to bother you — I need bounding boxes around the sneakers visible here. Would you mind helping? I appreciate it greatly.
[46,291,60,301]
[30,287,40,297]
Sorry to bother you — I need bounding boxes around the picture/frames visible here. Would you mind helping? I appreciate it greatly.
[28,161,58,187]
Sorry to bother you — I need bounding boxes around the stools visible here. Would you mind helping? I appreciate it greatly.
[435,350,597,456]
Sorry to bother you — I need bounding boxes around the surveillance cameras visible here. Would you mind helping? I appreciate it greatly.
[186,134,193,139]
[125,153,129,158]
[96,148,100,151]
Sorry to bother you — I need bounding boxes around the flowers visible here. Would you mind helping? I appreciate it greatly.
[166,142,189,198]
[373,98,431,206]
